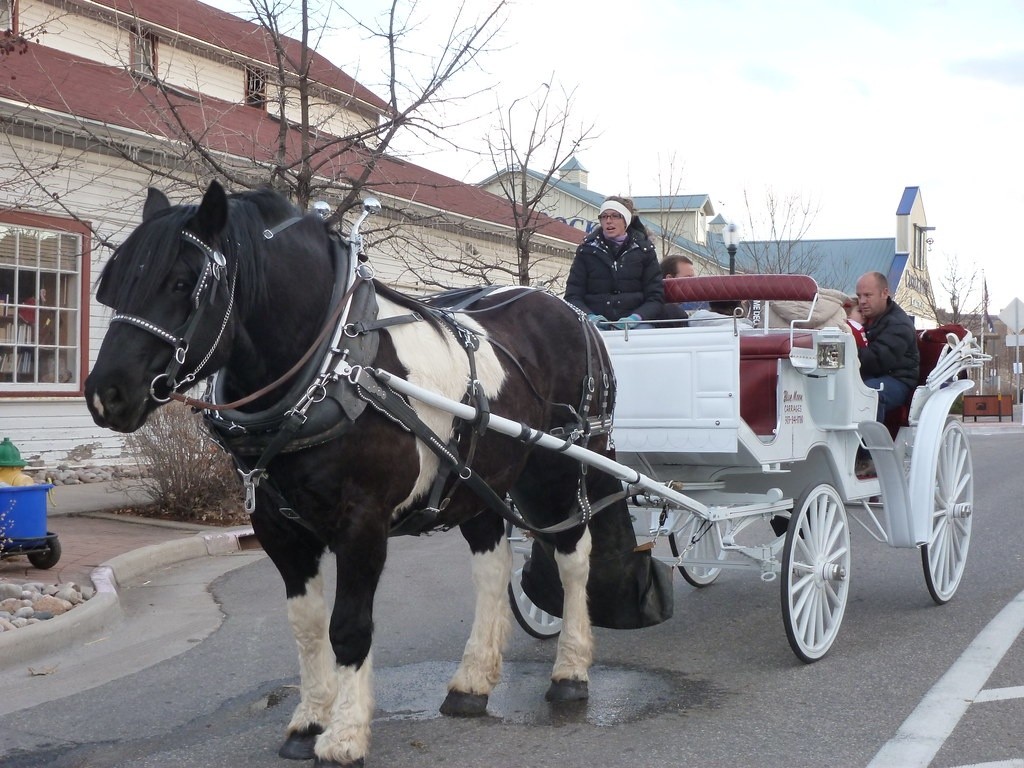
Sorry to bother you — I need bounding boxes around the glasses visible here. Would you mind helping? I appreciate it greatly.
[598,213,623,220]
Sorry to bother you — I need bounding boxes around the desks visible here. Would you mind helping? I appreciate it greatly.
[0,314,35,381]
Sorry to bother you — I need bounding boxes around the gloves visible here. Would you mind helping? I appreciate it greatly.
[615,314,639,331]
[586,314,610,330]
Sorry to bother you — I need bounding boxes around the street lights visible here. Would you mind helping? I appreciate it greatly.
[719,222,739,279]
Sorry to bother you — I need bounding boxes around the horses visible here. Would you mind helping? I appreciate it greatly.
[81,179,619,768]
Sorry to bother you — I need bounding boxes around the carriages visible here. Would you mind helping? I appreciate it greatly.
[76,181,995,768]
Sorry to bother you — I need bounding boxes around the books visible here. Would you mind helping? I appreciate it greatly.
[0,300,32,372]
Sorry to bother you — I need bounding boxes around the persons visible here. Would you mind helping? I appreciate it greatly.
[755,272,919,476]
[565,196,665,330]
[660,255,753,329]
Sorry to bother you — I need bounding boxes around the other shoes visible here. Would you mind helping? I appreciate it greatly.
[856,458,874,475]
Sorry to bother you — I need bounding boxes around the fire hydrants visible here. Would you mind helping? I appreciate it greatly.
[0,437,36,487]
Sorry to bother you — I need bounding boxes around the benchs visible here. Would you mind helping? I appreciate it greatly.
[663,272,819,353]
[882,329,955,420]
[748,326,881,431]
